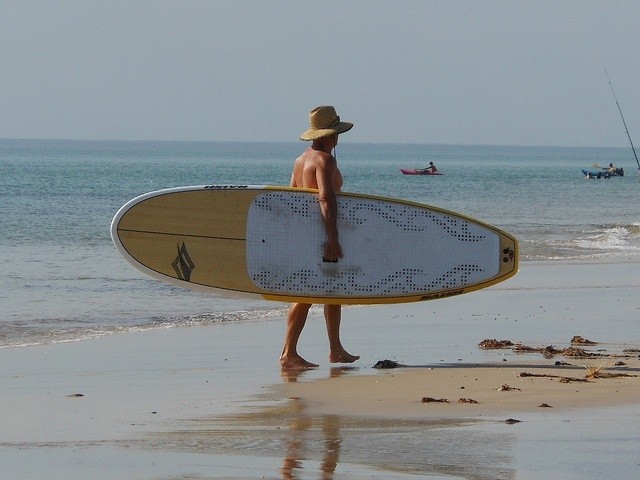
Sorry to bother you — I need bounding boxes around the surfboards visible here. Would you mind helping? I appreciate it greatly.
[110,184,518,305]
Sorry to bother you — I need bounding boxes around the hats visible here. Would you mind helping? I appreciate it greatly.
[300,105,353,141]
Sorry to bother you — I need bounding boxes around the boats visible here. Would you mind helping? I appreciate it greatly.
[401,167,444,175]
[582,168,624,178]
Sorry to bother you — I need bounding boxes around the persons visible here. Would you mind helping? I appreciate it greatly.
[280,104,359,369]
[422,161,436,173]
[609,162,615,174]
